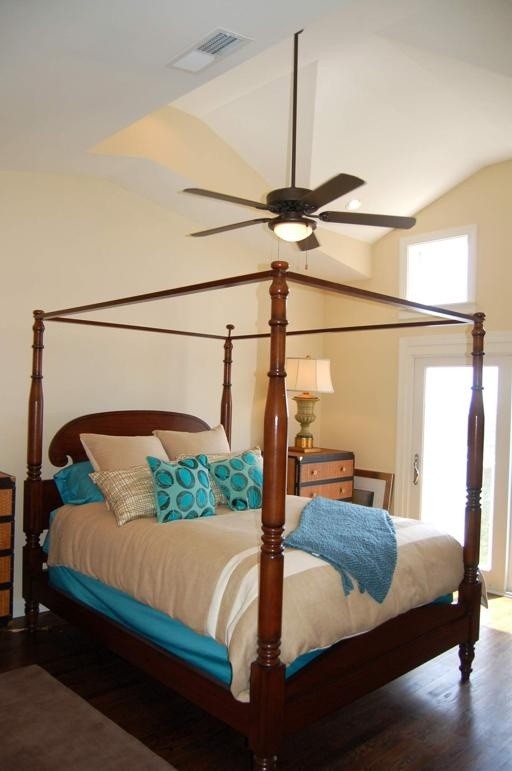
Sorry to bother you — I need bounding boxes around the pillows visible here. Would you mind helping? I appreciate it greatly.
[54,425,263,527]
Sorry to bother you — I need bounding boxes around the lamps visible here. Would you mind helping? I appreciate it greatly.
[284,355,334,453]
[263,211,317,243]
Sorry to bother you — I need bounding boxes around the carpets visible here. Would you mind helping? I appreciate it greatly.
[0,662,171,769]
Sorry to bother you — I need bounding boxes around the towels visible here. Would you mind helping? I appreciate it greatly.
[284,495,397,604]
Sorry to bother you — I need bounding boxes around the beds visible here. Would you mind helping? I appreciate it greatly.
[22,261,488,770]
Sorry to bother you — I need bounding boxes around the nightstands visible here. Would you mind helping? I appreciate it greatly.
[0,470,15,622]
[287,447,354,503]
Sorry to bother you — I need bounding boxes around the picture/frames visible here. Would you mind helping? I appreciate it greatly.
[352,470,395,511]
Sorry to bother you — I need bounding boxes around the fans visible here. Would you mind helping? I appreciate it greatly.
[181,32,416,250]
